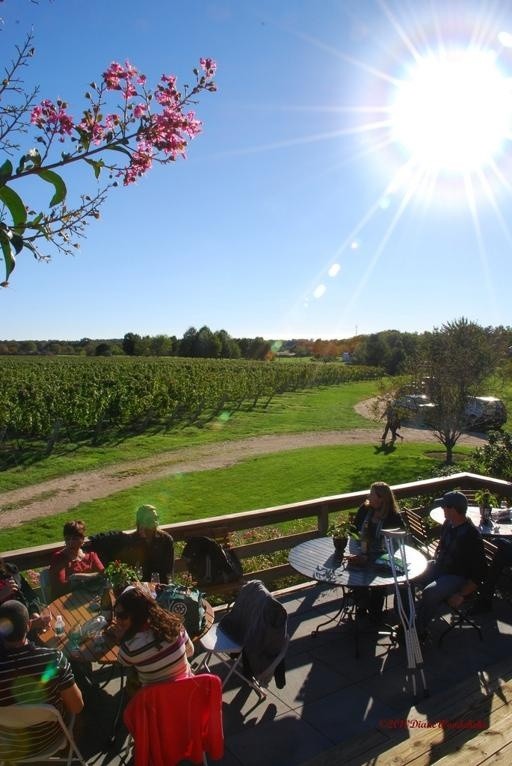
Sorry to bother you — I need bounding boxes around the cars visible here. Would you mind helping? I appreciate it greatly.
[391,394,441,427]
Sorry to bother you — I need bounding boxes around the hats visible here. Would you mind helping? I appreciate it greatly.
[434,491,467,510]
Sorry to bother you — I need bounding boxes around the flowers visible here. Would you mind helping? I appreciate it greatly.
[321,514,361,541]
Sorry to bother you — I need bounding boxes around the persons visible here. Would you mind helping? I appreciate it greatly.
[378,400,395,440]
[1,598,86,763]
[1,558,47,619]
[354,482,406,625]
[112,588,195,698]
[120,504,176,585]
[389,413,404,442]
[50,518,107,596]
[393,491,489,646]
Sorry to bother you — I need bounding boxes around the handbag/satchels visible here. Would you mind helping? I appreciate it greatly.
[154,583,207,636]
[184,536,229,584]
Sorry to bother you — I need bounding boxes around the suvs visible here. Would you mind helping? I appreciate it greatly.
[461,395,507,431]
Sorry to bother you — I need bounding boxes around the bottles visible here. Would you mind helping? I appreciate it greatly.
[53,614,66,638]
[93,631,104,654]
[360,518,372,555]
[68,624,83,652]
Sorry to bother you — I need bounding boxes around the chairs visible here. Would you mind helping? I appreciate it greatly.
[348,491,511,644]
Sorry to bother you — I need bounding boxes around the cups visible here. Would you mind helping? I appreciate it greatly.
[134,566,144,580]
[150,572,160,590]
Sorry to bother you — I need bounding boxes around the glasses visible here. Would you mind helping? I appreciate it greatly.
[116,611,129,620]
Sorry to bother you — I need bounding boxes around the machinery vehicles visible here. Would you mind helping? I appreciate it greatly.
[396,361,441,396]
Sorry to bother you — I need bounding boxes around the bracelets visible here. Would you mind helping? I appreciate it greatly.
[457,591,465,598]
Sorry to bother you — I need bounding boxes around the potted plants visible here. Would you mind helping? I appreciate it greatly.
[331,536,348,554]
